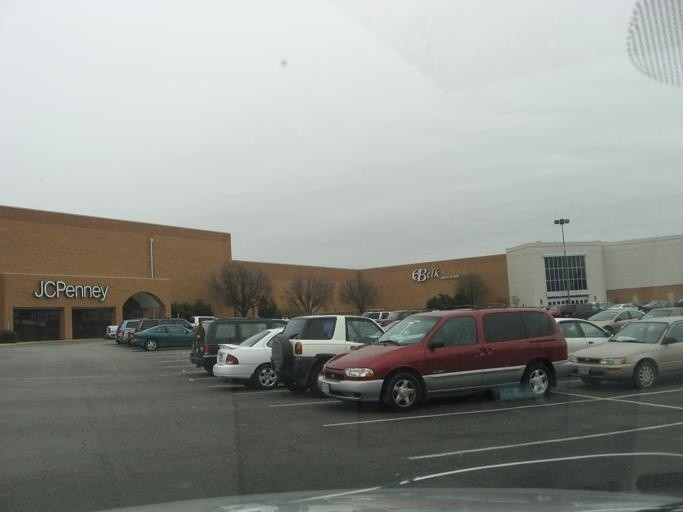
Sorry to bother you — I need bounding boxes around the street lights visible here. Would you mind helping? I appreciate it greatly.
[551,219,574,301]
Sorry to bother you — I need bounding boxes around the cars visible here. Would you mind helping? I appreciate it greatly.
[46,316,59,325]
[101,312,219,352]
[14,316,45,329]
